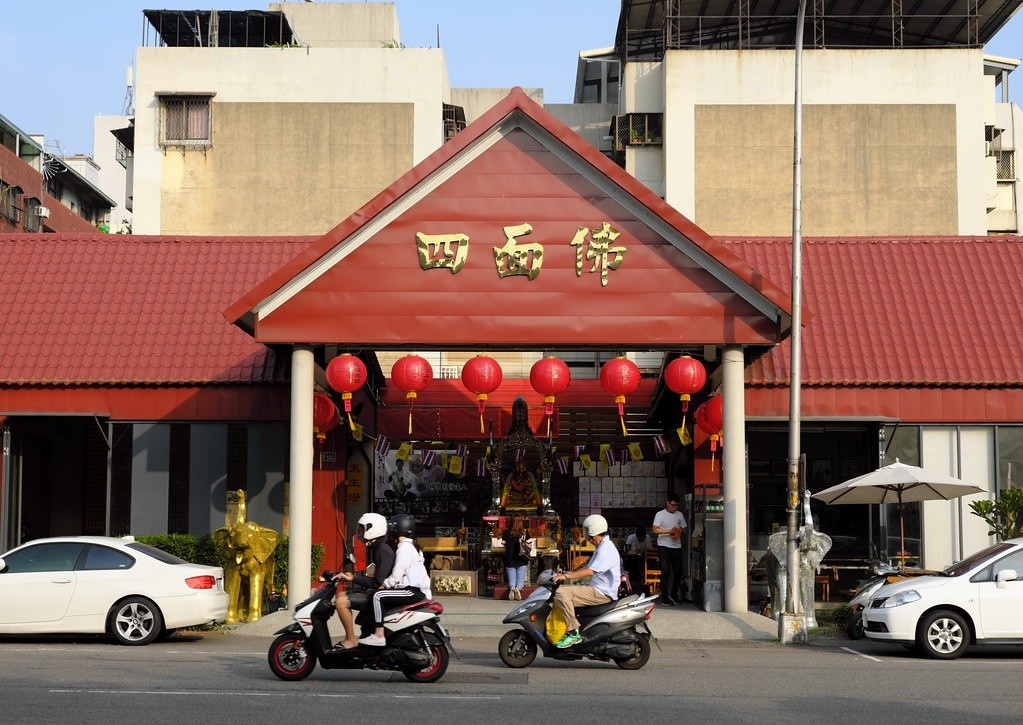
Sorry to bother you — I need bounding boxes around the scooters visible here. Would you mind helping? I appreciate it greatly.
[499,559,664,671]
[846,542,961,641]
[267,551,460,684]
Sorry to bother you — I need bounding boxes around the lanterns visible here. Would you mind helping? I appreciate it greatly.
[325,354,368,432]
[391,354,433,434]
[313,394,335,433]
[461,352,502,433]
[598,357,640,437]
[528,355,571,437]
[664,356,700,436]
[696,395,723,472]
[316,406,341,469]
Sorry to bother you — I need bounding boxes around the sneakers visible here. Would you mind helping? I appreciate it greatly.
[555,629,583,648]
[359,634,386,646]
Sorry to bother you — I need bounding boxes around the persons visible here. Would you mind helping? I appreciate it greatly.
[330,514,396,653]
[550,516,620,649]
[625,528,652,556]
[358,514,432,647]
[653,494,687,605]
[502,513,532,600]
[384,456,412,500]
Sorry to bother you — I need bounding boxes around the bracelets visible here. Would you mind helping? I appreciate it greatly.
[564,574,568,579]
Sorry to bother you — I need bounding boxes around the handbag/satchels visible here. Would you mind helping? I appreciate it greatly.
[518,535,531,563]
[545,597,567,644]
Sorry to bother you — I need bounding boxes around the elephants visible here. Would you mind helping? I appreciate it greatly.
[767,489,833,631]
[212,488,280,623]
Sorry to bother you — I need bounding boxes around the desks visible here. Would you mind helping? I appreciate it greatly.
[816,575,830,602]
[481,550,560,596]
[423,545,468,572]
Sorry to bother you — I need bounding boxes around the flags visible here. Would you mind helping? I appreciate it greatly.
[346,413,693,477]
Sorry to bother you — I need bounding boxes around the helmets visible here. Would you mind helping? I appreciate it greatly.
[583,514,608,537]
[358,513,388,540]
[387,514,415,534]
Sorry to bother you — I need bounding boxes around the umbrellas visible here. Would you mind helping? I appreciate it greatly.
[808,458,989,568]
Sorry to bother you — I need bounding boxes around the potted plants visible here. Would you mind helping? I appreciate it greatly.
[631,128,655,142]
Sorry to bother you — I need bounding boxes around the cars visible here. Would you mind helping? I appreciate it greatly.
[862,536,1023,661]
[0,535,231,646]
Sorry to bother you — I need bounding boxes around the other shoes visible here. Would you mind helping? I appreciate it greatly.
[514,587,521,600]
[669,594,680,604]
[509,588,514,600]
[662,600,674,606]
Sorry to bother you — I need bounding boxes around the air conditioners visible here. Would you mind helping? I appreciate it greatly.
[38,206,50,218]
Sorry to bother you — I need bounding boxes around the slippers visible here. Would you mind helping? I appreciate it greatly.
[332,641,357,653]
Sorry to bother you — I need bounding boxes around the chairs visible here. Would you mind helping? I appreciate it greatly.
[645,547,662,597]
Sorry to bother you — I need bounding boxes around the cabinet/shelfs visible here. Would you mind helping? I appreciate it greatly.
[691,482,725,611]
